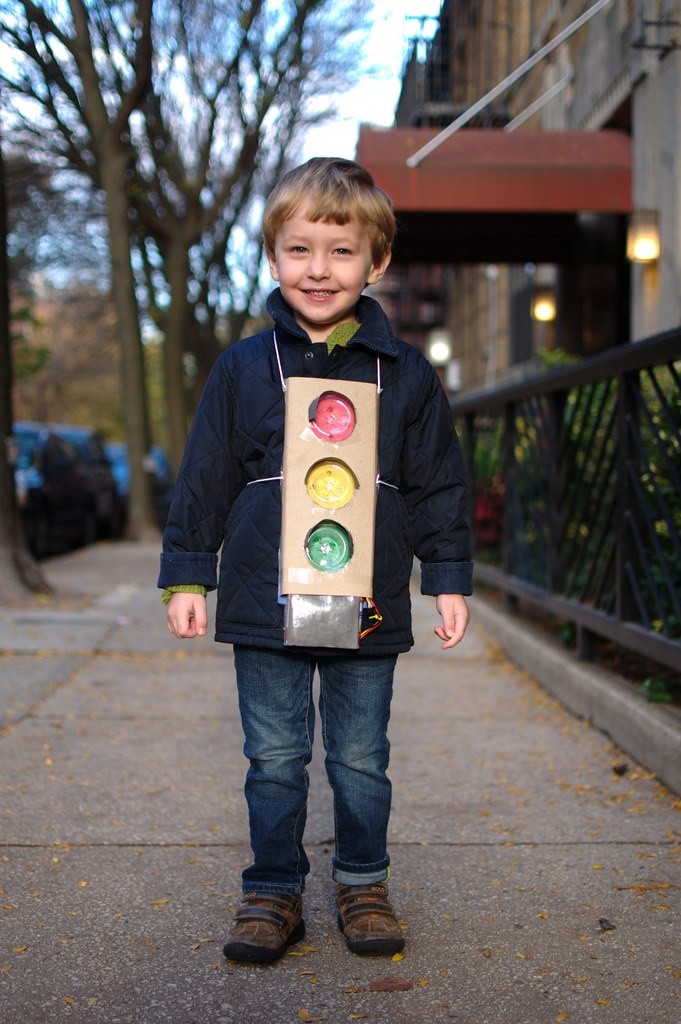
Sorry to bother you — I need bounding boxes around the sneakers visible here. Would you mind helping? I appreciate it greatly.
[222,892,305,963]
[334,882,405,955]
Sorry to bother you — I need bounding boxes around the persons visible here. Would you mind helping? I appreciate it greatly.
[156,158,472,965]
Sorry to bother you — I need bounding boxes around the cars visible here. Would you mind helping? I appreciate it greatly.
[102,442,171,517]
[11,426,96,559]
[55,429,121,542]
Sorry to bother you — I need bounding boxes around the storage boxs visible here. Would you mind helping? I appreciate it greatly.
[280,377,379,598]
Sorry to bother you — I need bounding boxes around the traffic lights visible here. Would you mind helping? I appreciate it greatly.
[278,376,378,605]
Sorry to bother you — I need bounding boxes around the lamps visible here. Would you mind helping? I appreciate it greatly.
[529,285,557,323]
[625,208,661,265]
